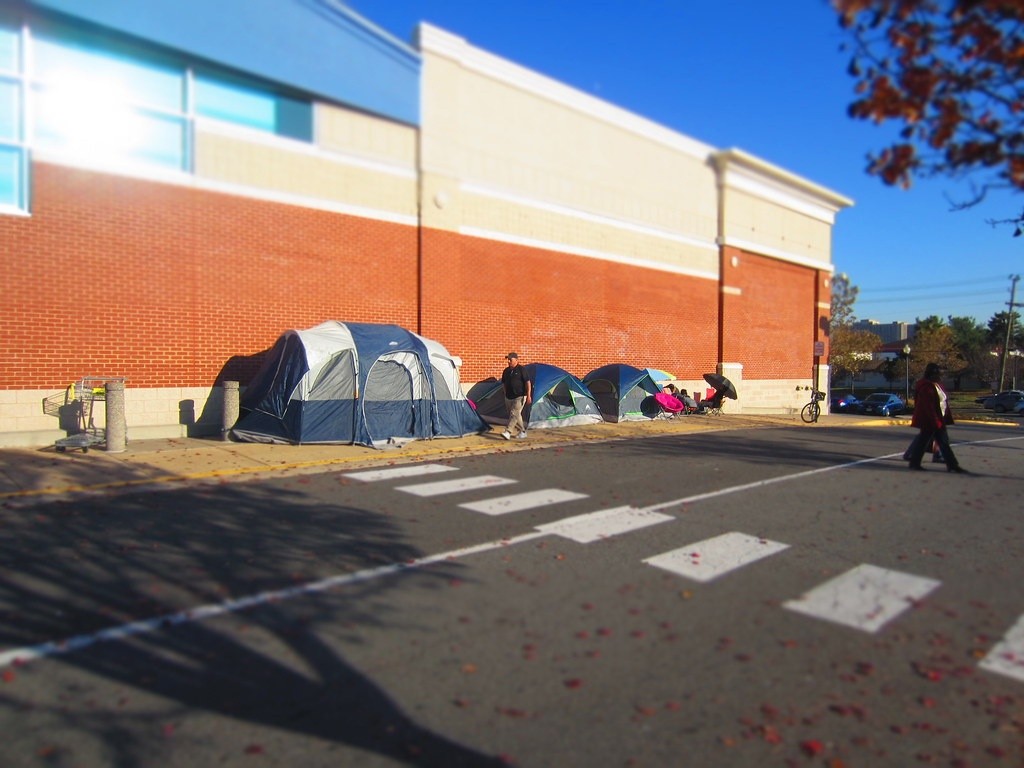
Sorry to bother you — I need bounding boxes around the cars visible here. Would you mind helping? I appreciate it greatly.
[1014,398,1024,416]
[984,390,1024,413]
[856,393,903,418]
[830,393,862,414]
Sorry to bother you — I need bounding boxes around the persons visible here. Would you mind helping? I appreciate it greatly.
[501,352,532,440]
[662,383,698,415]
[696,384,726,413]
[902,362,966,473]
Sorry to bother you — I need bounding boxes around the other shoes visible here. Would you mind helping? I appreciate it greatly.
[947,466,967,473]
[910,464,923,470]
[931,453,945,463]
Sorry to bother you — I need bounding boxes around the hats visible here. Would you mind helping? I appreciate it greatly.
[505,353,518,358]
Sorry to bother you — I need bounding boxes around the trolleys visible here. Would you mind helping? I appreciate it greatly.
[42,376,126,453]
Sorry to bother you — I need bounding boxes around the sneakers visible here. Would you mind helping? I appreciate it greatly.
[500,431,511,440]
[515,431,528,438]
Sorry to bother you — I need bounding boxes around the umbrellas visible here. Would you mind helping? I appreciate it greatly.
[703,373,737,401]
[643,368,677,383]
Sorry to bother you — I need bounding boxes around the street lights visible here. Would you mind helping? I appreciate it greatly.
[903,343,910,405]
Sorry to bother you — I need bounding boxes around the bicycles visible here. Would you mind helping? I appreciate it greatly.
[800,387,826,423]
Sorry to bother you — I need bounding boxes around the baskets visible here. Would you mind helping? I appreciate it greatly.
[816,392,826,401]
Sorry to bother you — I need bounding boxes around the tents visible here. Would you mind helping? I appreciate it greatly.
[231,318,496,452]
[474,363,605,431]
[580,362,667,424]
